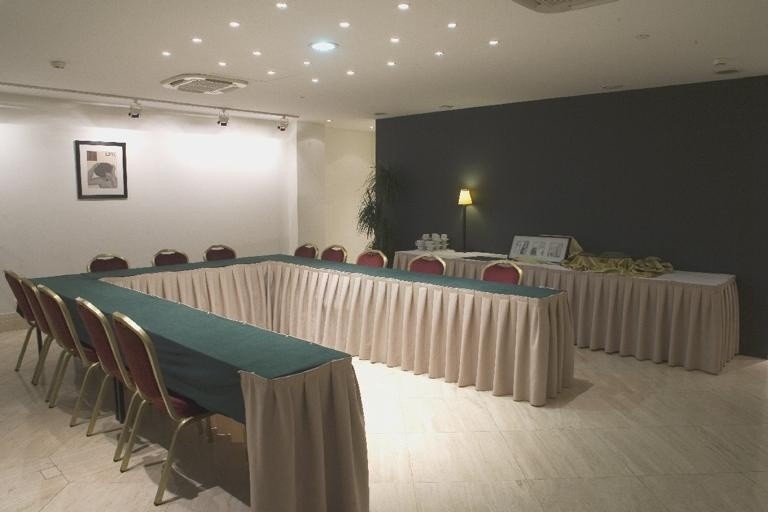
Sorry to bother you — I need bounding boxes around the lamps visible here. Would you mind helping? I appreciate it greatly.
[127,103,140,116]
[217,110,228,125]
[306,40,340,54]
[276,114,288,132]
[458,189,474,253]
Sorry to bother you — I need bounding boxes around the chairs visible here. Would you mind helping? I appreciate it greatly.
[37,284,97,407]
[408,254,445,276]
[321,245,347,262]
[357,250,388,267]
[74,296,137,461]
[294,243,317,258]
[112,311,216,506]
[20,278,67,403]
[3,268,46,382]
[480,260,522,286]
[151,249,188,266]
[86,254,129,271]
[202,244,236,262]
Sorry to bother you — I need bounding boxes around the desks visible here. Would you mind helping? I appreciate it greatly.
[23,253,575,512]
[393,249,740,375]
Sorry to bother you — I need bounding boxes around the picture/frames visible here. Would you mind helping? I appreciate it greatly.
[73,139,129,201]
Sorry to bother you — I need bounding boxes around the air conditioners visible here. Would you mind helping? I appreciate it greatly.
[161,74,248,96]
